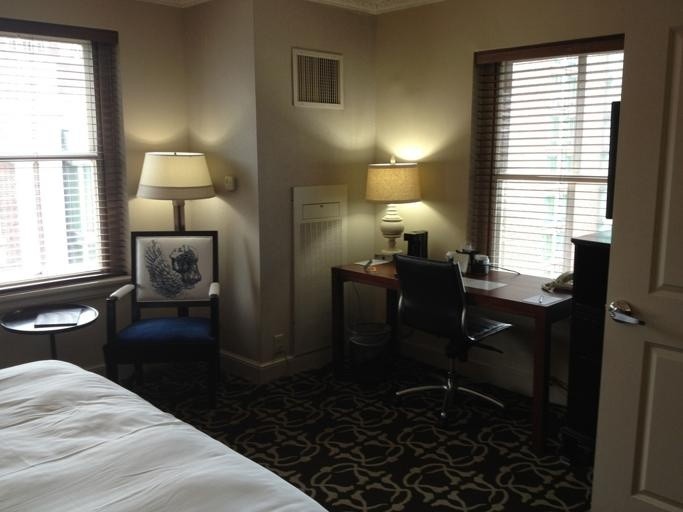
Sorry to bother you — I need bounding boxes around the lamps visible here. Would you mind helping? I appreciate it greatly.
[366,164,423,256]
[133,151,217,231]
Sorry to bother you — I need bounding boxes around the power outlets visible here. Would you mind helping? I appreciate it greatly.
[274,334,288,353]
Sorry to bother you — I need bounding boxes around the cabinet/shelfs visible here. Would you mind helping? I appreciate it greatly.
[570,233,613,469]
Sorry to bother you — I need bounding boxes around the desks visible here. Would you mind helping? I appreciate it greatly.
[1,303,101,360]
[331,259,573,456]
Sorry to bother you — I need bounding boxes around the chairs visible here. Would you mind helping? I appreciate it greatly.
[103,230,224,409]
[391,255,516,427]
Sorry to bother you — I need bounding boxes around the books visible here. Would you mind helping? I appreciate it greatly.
[33,307,82,326]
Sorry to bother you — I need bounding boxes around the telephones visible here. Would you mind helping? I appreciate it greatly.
[553,271,573,290]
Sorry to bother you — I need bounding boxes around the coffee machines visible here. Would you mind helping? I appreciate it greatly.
[404,229,428,259]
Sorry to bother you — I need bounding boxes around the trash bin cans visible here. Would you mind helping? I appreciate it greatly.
[345,320,392,389]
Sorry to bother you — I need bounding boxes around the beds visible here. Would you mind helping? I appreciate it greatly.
[1,360,332,512]
